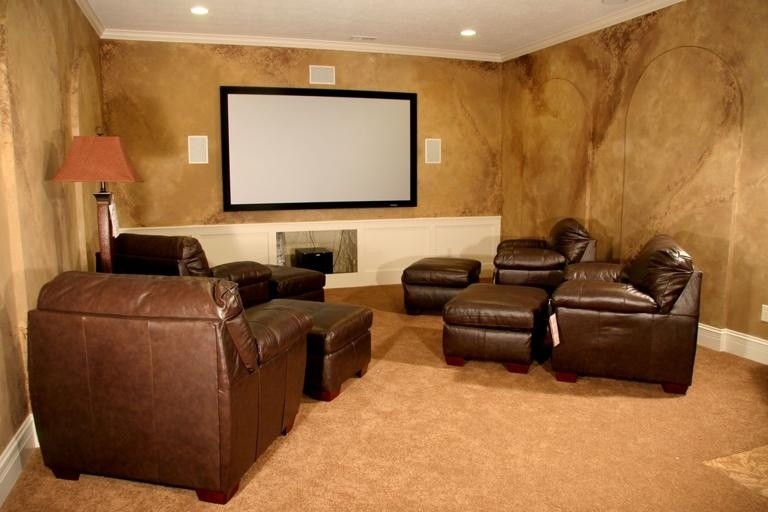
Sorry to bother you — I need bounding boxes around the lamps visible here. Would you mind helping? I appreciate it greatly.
[50,132,140,274]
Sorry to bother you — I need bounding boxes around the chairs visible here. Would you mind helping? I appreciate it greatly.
[114,227,272,306]
[494,217,597,285]
[547,233,704,395]
[27,274,313,506]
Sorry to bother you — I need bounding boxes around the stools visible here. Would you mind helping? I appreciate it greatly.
[244,299,372,401]
[442,284,548,373]
[401,257,480,316]
[266,263,326,301]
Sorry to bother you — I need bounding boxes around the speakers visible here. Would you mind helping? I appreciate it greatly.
[296,247,333,273]
